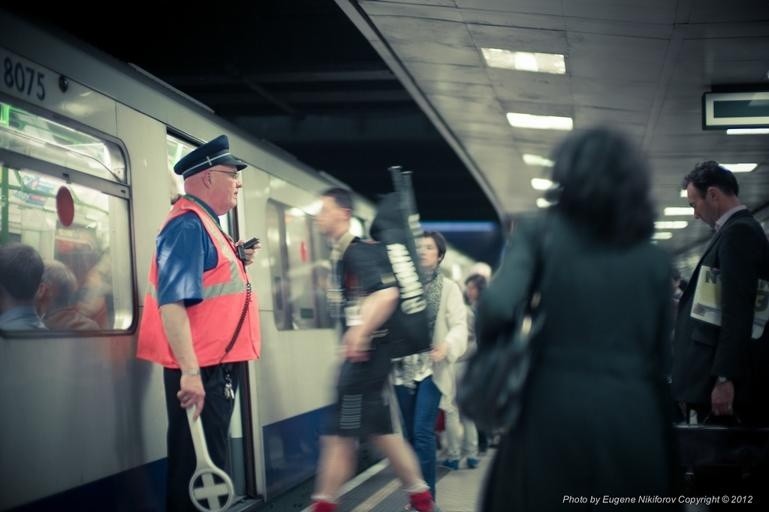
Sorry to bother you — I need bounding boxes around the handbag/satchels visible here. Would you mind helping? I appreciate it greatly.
[453,349,518,428]
[675,426,768,477]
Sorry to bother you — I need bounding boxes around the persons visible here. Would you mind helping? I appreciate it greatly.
[133,131,265,510]
[472,122,689,509]
[294,186,440,511]
[2,189,348,331]
[438,301,481,472]
[478,273,496,456]
[411,229,462,503]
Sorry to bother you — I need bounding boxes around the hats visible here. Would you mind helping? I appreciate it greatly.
[173,132,249,180]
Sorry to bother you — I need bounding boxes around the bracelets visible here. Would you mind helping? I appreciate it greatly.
[718,377,730,384]
[179,368,202,377]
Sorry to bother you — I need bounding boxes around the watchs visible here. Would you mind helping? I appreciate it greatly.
[673,159,769,510]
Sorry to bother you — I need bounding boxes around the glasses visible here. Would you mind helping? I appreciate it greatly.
[207,168,241,178]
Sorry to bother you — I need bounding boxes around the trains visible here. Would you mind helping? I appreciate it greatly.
[0,10,474,512]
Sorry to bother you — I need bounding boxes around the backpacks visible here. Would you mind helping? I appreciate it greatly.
[345,193,433,357]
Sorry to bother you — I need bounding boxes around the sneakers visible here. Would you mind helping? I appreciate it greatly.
[436,456,481,472]
